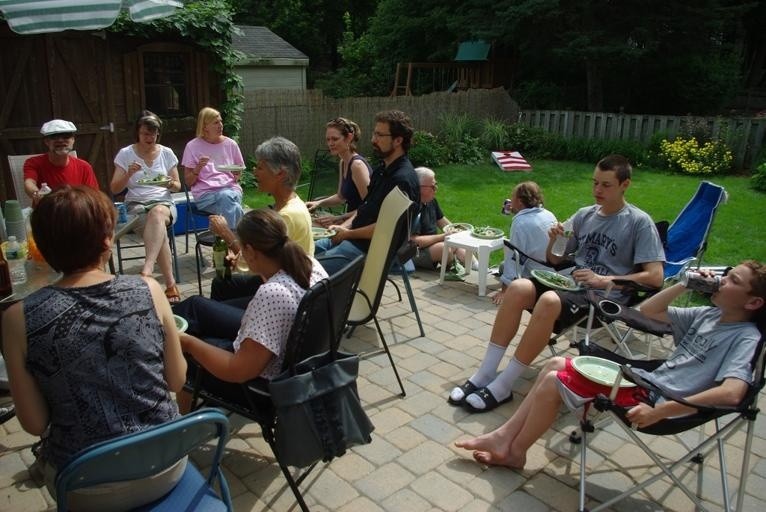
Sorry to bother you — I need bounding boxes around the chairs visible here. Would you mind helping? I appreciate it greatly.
[180,253,366,512]
[55,408,236,512]
[346,185,416,397]
[4,151,78,207]
[305,145,345,205]
[502,218,671,362]
[179,163,223,270]
[659,177,726,285]
[390,226,427,338]
[569,299,766,512]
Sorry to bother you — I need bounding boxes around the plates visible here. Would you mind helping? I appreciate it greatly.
[471,227,505,239]
[570,355,638,388]
[311,227,337,239]
[136,176,174,187]
[441,222,472,235]
[215,165,247,173]
[529,270,584,293]
[172,314,189,335]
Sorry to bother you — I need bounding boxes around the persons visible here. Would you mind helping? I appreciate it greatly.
[305,117,373,227]
[23,119,99,208]
[181,107,247,230]
[110,110,181,304]
[313,110,422,280]
[455,258,766,470]
[492,180,576,306]
[392,167,479,274]
[448,154,666,414]
[209,137,315,310]
[1,185,188,512]
[171,208,329,418]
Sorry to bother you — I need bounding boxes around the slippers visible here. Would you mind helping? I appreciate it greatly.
[461,386,515,412]
[448,379,486,406]
[165,283,180,304]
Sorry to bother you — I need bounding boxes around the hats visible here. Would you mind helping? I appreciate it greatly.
[39,119,77,139]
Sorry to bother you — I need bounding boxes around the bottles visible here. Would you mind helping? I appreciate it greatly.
[213,213,232,280]
[114,202,127,224]
[38,183,51,202]
[5,236,27,285]
[681,271,721,294]
[551,213,576,257]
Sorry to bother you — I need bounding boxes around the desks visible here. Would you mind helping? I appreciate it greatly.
[439,228,512,298]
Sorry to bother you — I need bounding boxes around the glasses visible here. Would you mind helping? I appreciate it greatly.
[371,129,394,137]
[419,183,439,190]
[328,118,352,131]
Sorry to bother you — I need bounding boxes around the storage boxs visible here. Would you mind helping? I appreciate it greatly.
[169,191,214,236]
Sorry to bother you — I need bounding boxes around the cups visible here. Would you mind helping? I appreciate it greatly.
[4,199,25,243]
